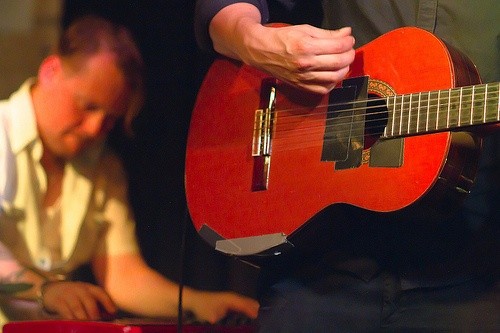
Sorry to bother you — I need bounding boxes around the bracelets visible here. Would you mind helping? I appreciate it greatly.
[36,273,73,319]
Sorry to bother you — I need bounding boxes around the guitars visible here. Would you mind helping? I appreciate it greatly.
[184,22,500,270]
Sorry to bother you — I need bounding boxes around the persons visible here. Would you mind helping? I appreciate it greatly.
[194,0,500,333]
[1,16,260,322]
